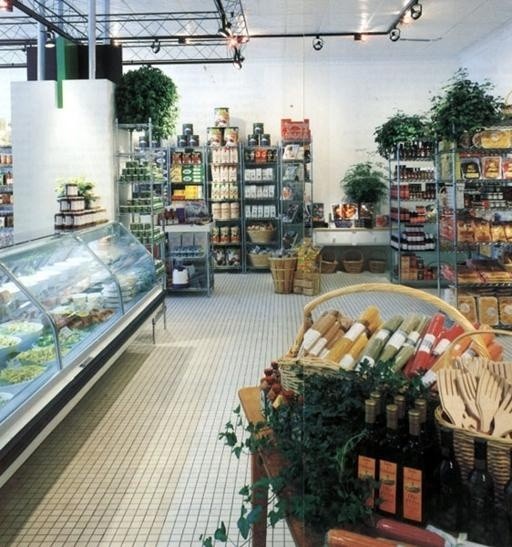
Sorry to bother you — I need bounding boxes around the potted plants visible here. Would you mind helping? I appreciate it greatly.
[339,160,388,228]
[52,176,101,209]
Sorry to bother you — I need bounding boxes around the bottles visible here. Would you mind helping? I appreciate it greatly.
[390,224,436,251]
[409,211,418,224]
[399,140,433,161]
[394,165,435,182]
[352,386,511,546]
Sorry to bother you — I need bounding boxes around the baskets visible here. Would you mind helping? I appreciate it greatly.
[342,249,365,272]
[246,249,270,266]
[272,283,495,400]
[267,255,298,269]
[248,225,276,242]
[431,330,511,506]
[271,270,293,294]
[320,248,337,273]
[367,249,388,272]
[293,267,321,295]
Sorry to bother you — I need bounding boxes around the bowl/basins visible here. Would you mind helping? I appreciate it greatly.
[8,257,89,302]
[14,323,44,350]
[0,335,22,361]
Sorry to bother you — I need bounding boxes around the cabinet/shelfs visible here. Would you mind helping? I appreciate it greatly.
[432,118,512,337]
[0,146,13,230]
[113,117,313,297]
[387,142,474,290]
[0,219,165,489]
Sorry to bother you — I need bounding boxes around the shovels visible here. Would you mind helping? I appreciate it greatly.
[436,357,512,439]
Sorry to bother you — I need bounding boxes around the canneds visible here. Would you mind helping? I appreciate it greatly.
[176,124,199,147]
[248,123,270,146]
[139,131,160,147]
[54,184,106,229]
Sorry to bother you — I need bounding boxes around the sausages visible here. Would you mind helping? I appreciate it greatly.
[376,518,445,547]
[326,529,398,547]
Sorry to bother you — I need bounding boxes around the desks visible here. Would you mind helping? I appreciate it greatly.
[236,382,325,547]
